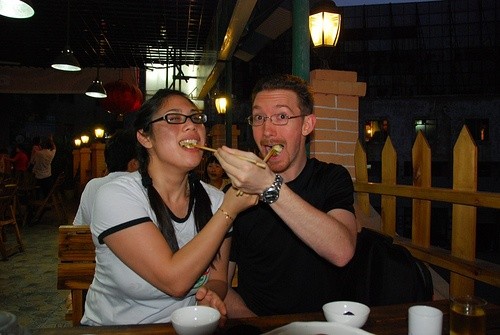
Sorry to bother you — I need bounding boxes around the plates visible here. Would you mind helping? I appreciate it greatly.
[258,321,376,335]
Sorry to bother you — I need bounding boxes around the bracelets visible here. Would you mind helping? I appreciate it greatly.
[217,207,235,225]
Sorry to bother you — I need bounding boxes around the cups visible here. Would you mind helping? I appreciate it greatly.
[448,295,488,335]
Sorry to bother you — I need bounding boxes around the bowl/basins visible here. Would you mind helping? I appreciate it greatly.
[322,300,371,330]
[171,305,221,335]
[0,309,30,335]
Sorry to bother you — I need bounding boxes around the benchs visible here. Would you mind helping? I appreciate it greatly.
[30,171,67,226]
[57,225,237,326]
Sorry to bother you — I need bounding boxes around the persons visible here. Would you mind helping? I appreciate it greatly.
[0,134,61,204]
[213,74,368,319]
[72,126,144,227]
[203,156,231,190]
[78,88,235,328]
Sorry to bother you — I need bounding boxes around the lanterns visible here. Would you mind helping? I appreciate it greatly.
[97,79,145,115]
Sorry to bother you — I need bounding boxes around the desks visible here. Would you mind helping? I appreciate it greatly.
[24,297,500,335]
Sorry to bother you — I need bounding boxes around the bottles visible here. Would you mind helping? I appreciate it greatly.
[407,306,444,335]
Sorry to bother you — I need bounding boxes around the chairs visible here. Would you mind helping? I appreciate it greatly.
[0,184,24,261]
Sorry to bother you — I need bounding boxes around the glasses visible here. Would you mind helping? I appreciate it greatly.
[148,113,207,125]
[246,113,308,126]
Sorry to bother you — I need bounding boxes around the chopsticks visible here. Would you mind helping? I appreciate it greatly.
[190,143,258,165]
[237,146,277,197]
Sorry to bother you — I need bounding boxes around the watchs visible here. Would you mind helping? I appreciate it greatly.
[258,174,283,204]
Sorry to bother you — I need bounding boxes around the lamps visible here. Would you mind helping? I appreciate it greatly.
[0,0,36,20]
[50,0,82,71]
[84,36,108,98]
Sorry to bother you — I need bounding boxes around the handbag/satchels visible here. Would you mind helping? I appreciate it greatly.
[331,227,434,308]
